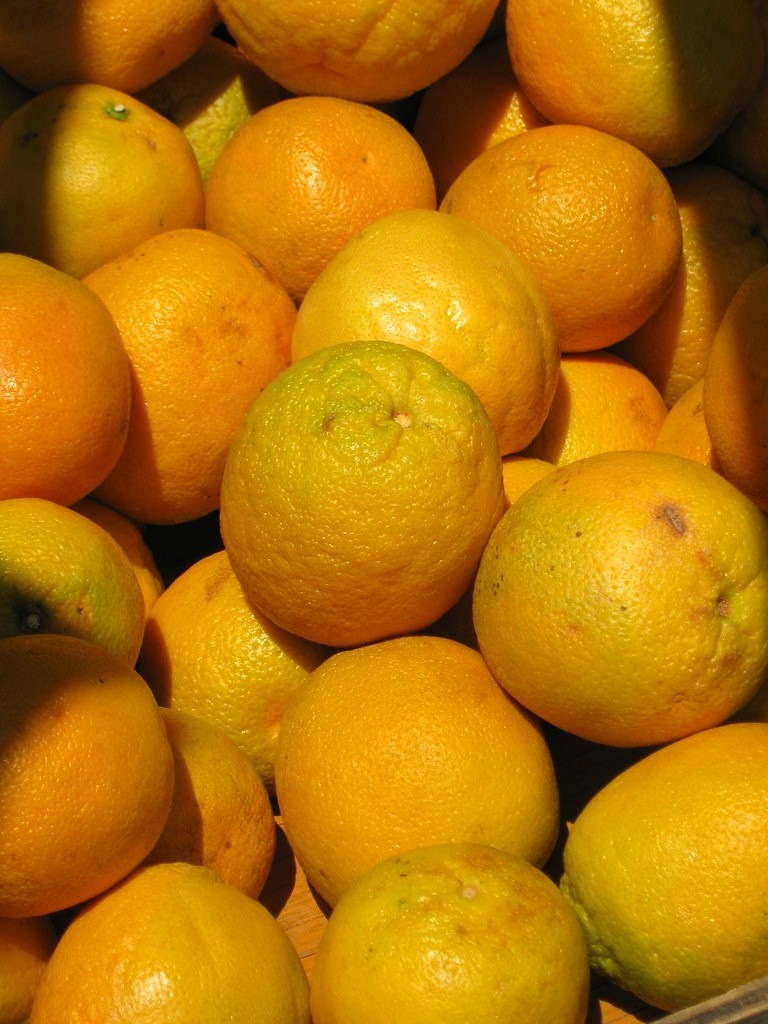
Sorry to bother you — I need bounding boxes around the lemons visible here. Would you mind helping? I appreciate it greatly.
[0,0,768,1024]
